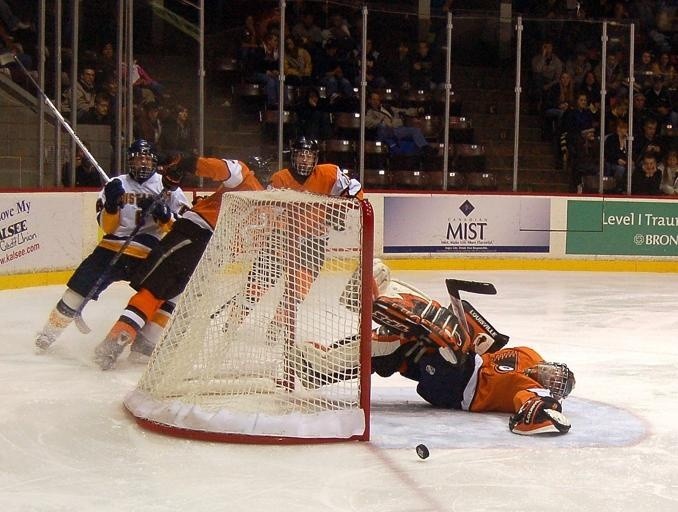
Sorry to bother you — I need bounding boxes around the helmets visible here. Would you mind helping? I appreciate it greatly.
[288,127,320,180]
[128,139,160,182]
[522,360,575,401]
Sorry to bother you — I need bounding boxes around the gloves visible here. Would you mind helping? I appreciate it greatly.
[148,148,198,177]
[137,194,170,222]
[104,178,125,214]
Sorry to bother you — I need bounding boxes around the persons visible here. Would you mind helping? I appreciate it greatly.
[290,259,575,436]
[29,138,193,363]
[220,136,364,347]
[93,156,278,371]
[1,1,676,194]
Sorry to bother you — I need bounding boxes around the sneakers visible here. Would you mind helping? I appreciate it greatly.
[130,337,157,356]
[91,352,112,371]
[35,336,51,351]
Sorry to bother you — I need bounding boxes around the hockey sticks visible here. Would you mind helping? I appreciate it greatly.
[0,52,110,183]
[74,184,167,334]
[446,278,497,335]
